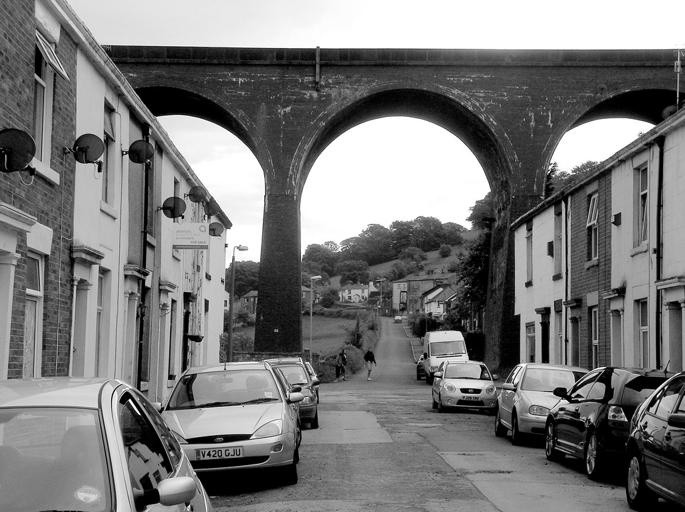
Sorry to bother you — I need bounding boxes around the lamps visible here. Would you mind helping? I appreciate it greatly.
[0,128,224,237]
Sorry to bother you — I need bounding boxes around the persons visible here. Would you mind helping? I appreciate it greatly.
[334,349,348,382]
[363,346,376,381]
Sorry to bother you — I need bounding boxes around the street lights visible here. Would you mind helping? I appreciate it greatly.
[377,278,386,302]
[308,275,323,358]
[463,284,471,329]
[226,241,250,363]
[360,283,366,302]
[410,261,416,276]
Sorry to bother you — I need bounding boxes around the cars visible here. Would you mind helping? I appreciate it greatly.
[494,363,590,446]
[152,360,305,489]
[1,376,214,512]
[402,316,407,320]
[393,315,403,325]
[270,364,304,434]
[414,355,426,380]
[302,360,321,403]
[431,361,499,418]
[544,360,679,481]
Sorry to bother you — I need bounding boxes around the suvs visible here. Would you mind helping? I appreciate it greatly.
[623,372,684,512]
[260,356,321,431]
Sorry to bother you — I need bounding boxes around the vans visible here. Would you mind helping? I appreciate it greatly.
[420,330,470,386]
